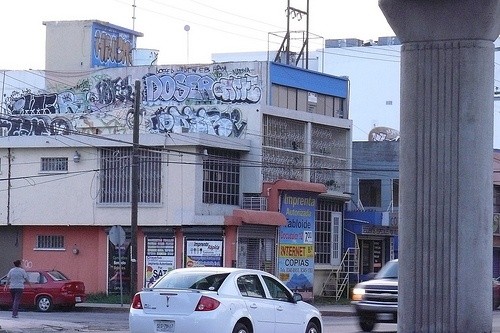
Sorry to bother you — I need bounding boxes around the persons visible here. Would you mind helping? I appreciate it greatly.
[2,260,32,318]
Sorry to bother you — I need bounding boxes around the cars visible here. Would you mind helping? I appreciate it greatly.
[350,258,398,332]
[129,267,324,333]
[0,270,87,313]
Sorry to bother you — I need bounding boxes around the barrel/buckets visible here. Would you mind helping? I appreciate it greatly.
[130,48,158,65]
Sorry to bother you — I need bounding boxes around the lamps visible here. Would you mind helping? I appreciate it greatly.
[199,148,208,161]
[73,151,81,163]
